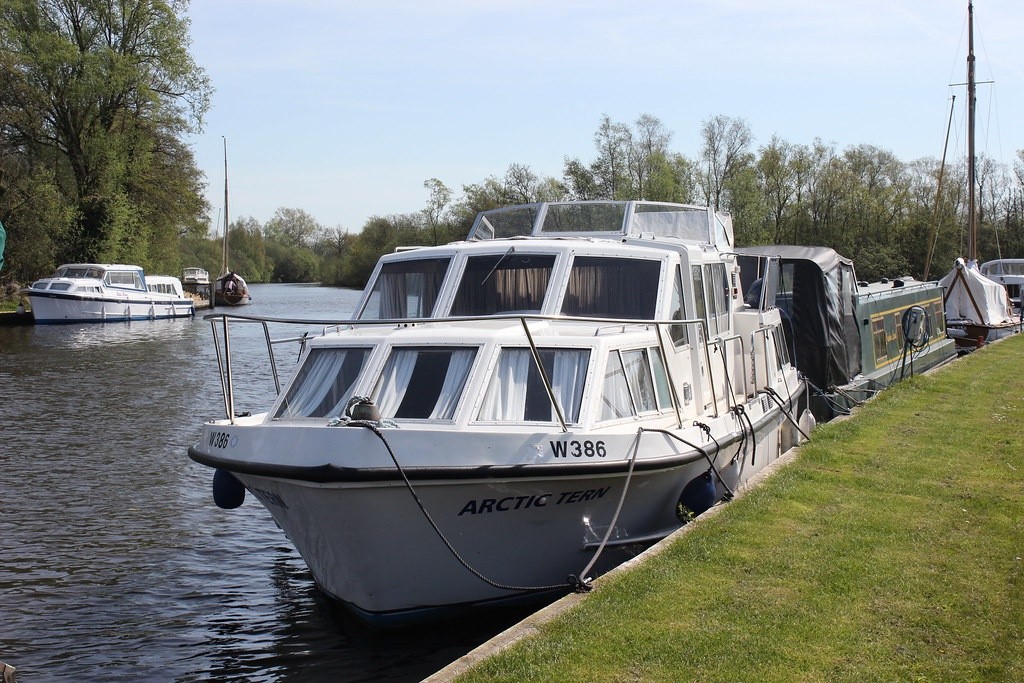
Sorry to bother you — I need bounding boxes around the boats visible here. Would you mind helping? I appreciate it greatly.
[214,138,251,306]
[921,0,1024,353]
[184,196,809,622]
[18,263,197,324]
[730,244,959,423]
[180,265,210,294]
